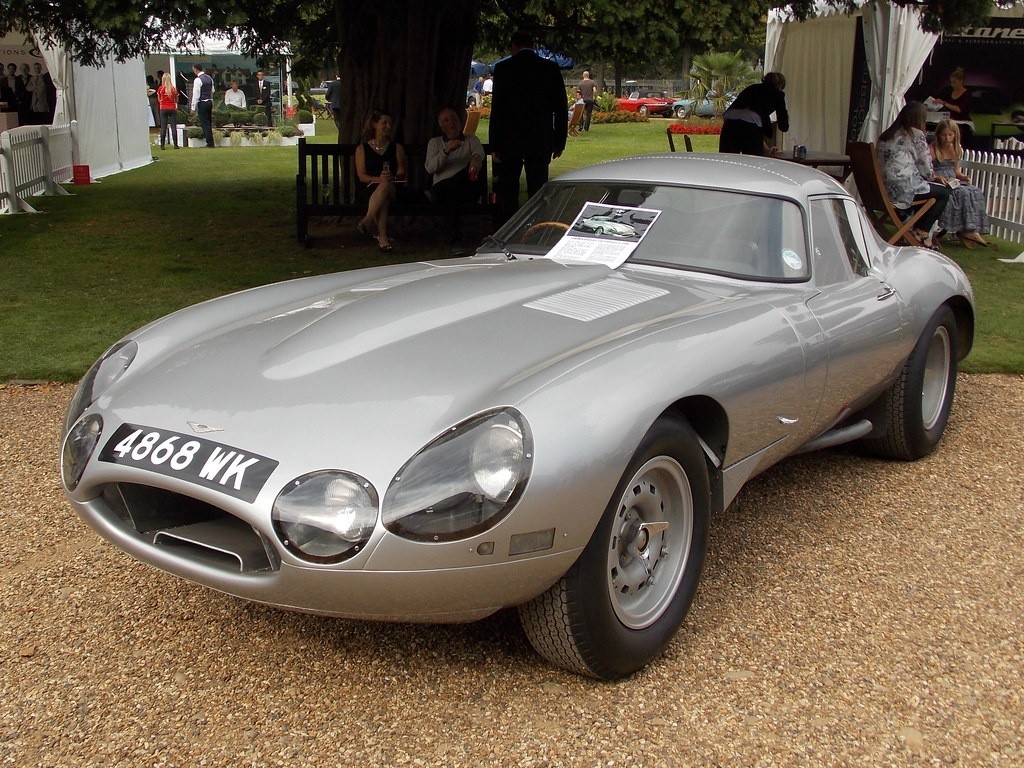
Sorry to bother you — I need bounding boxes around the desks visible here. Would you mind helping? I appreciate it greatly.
[772,151,851,184]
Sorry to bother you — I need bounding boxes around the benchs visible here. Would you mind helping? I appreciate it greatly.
[294,137,491,250]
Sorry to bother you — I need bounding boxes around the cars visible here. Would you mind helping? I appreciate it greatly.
[310,80,334,95]
[465,91,477,108]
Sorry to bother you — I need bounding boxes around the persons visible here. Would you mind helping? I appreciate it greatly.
[354,110,409,249]
[425,109,484,255]
[928,119,990,249]
[488,29,568,236]
[473,76,493,107]
[930,66,972,146]
[253,71,272,126]
[718,72,789,155]
[0,63,57,125]
[225,82,246,113]
[577,71,597,132]
[146,69,180,150]
[191,64,216,148]
[875,101,949,249]
[568,89,584,129]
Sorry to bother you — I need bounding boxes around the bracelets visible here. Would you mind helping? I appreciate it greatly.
[470,166,477,172]
[368,176,372,183]
[943,101,945,106]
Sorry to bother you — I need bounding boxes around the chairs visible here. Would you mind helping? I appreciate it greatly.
[569,103,585,136]
[846,139,942,252]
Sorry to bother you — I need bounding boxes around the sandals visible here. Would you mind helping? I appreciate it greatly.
[913,231,938,251]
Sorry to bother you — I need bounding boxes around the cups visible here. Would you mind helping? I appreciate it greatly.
[489,192,496,204]
[383,162,389,172]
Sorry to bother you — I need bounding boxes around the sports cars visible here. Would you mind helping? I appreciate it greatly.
[613,89,677,119]
[61,151,981,683]
[672,90,738,119]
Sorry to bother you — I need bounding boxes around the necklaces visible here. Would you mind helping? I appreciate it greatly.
[372,138,389,151]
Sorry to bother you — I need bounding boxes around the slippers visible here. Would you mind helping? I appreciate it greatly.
[960,229,988,247]
[956,231,976,250]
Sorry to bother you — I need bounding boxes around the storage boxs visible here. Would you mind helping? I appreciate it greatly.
[925,111,949,121]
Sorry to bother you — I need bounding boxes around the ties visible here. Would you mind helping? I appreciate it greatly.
[260,81,262,93]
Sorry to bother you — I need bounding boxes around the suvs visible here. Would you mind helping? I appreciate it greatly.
[263,75,299,96]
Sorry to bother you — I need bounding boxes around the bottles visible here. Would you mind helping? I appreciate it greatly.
[792,145,806,159]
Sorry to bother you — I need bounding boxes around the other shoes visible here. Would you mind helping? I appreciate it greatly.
[174,146,179,149]
[425,189,437,202]
[378,237,393,253]
[357,222,369,235]
[578,126,583,131]
[448,243,467,256]
[205,145,215,148]
[161,147,166,150]
